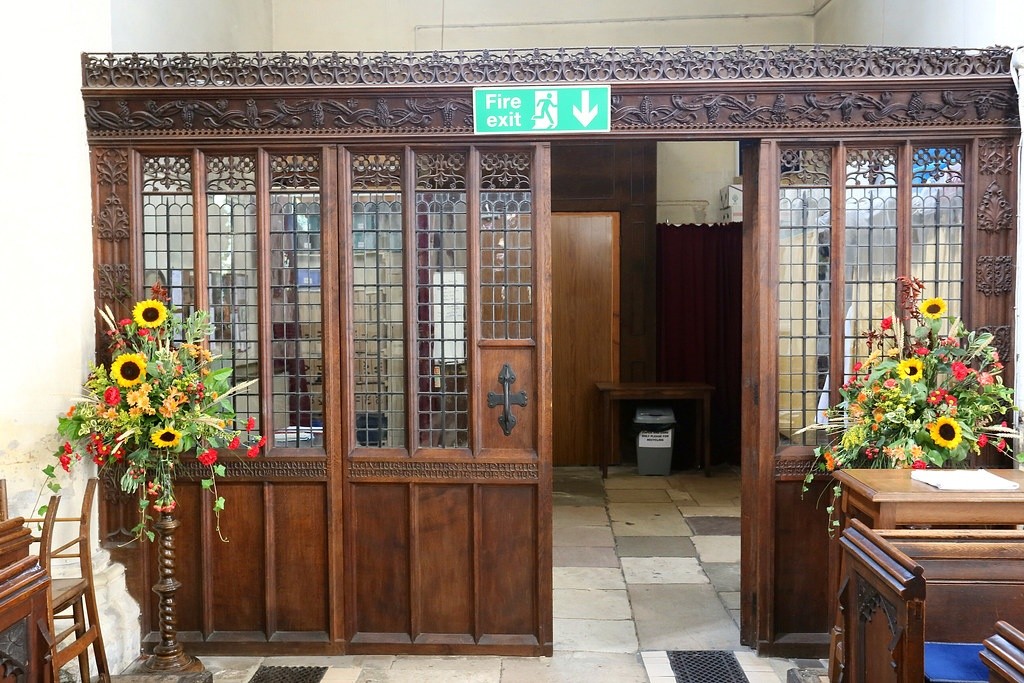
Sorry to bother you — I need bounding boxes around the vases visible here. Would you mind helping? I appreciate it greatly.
[141,478,205,675]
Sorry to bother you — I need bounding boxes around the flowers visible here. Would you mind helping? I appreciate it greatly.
[23,280,266,549]
[791,276,1024,543]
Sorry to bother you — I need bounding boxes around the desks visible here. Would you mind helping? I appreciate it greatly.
[595,382,716,479]
[828,469,1023,683]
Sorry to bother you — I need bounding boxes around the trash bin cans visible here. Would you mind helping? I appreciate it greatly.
[633,407,676,476]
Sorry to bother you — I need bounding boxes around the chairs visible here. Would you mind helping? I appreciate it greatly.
[0,478,111,683]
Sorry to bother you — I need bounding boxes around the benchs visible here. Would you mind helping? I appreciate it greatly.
[837,517,1024,682]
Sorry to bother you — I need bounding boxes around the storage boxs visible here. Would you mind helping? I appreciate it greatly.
[720,184,743,223]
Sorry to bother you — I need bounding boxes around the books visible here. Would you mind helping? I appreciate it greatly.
[911,468,1019,490]
[252,426,323,442]
